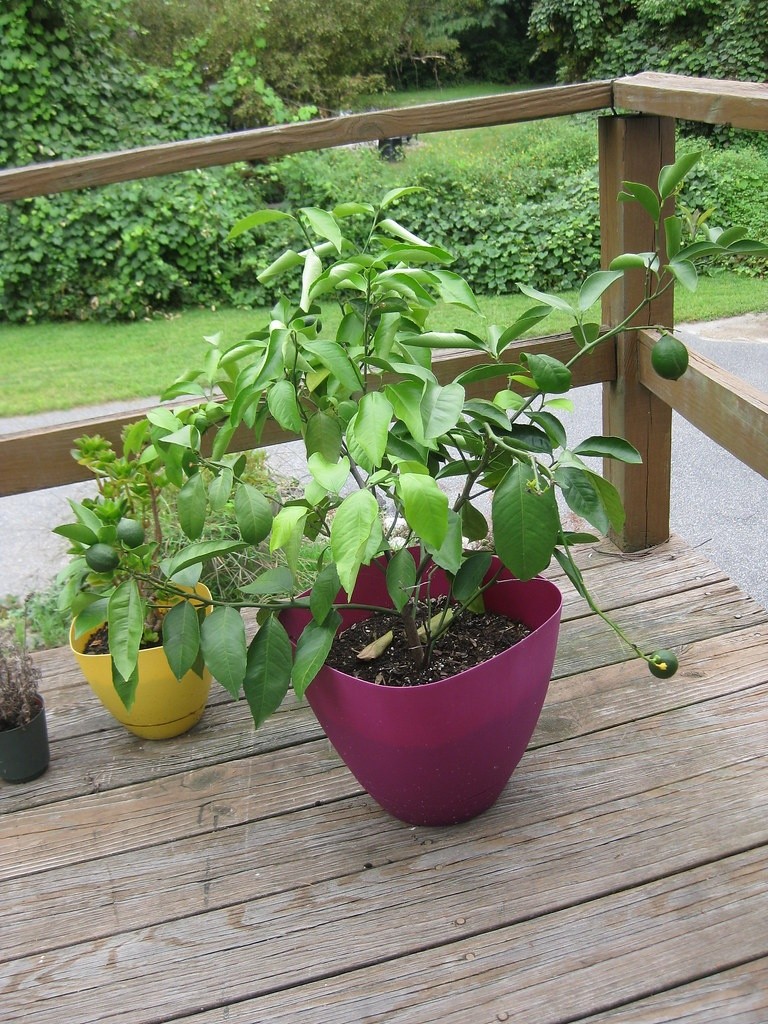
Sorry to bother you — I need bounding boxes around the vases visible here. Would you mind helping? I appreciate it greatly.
[0,692,50,784]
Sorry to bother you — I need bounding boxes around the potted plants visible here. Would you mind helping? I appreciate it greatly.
[48,149,767,829]
[62,419,316,741]
